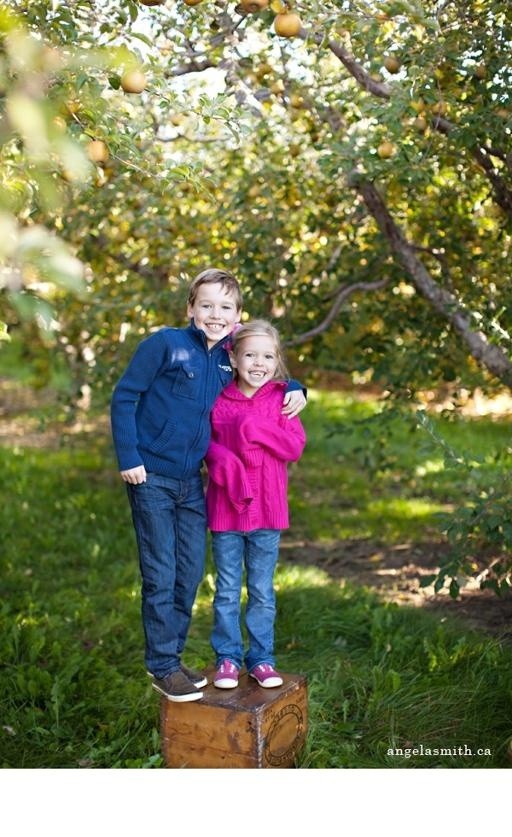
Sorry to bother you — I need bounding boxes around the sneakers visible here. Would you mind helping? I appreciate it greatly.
[145,659,207,689]
[152,668,204,703]
[212,656,241,689]
[247,659,284,690]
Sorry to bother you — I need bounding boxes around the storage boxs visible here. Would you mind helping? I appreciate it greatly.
[160,662,309,769]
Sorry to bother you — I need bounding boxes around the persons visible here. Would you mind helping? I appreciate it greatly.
[105,265,317,702]
[198,317,310,688]
